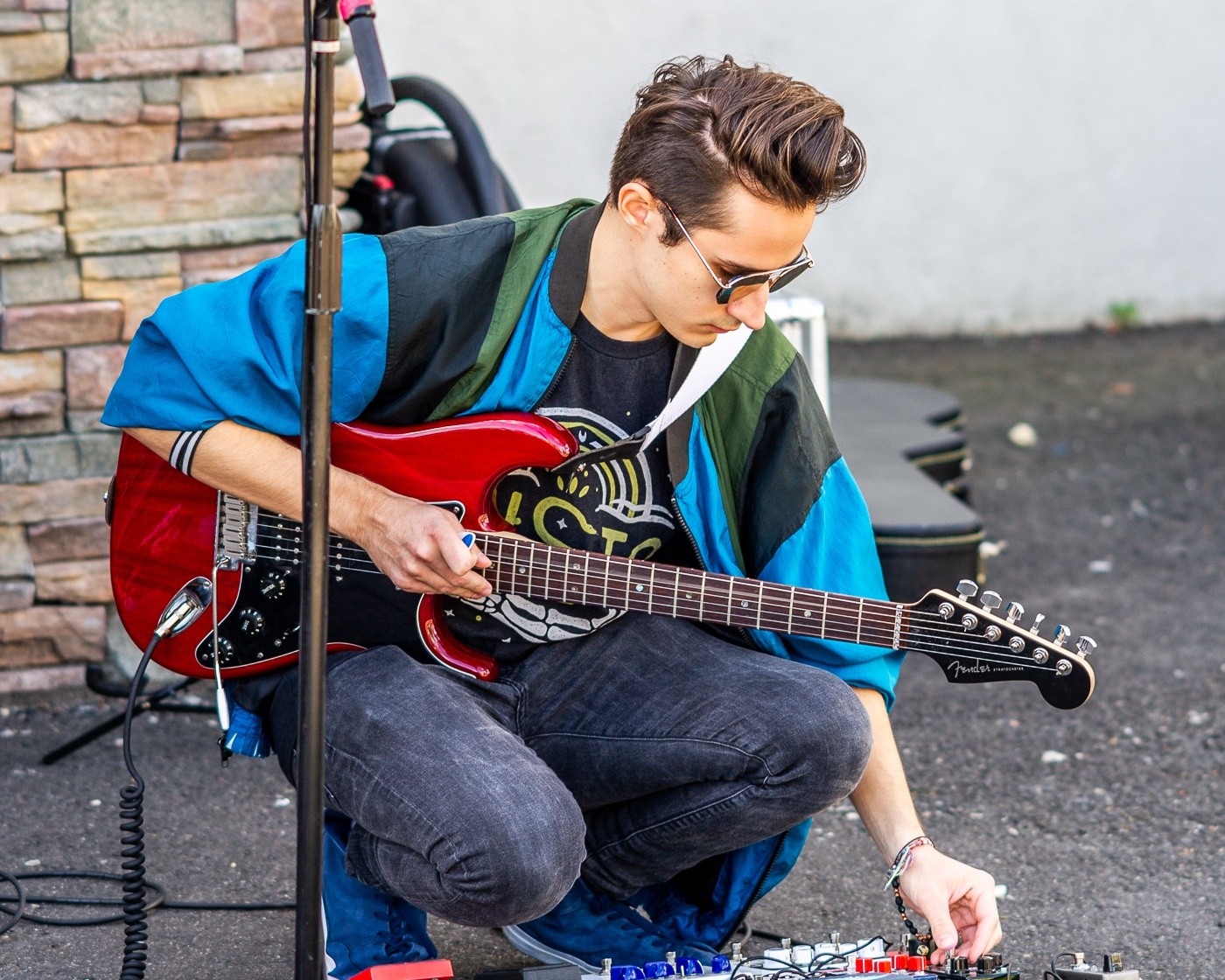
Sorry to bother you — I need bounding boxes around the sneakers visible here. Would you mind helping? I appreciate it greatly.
[318,819,438,980]
[501,870,727,980]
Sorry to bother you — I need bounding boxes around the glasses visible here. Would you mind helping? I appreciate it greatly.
[655,197,816,305]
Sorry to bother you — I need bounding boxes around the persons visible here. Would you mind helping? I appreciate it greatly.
[96,53,1006,980]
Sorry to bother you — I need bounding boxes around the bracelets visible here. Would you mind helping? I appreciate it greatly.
[886,836,934,957]
[169,430,208,477]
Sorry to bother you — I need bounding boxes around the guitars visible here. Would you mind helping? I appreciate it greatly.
[103,411,1095,767]
